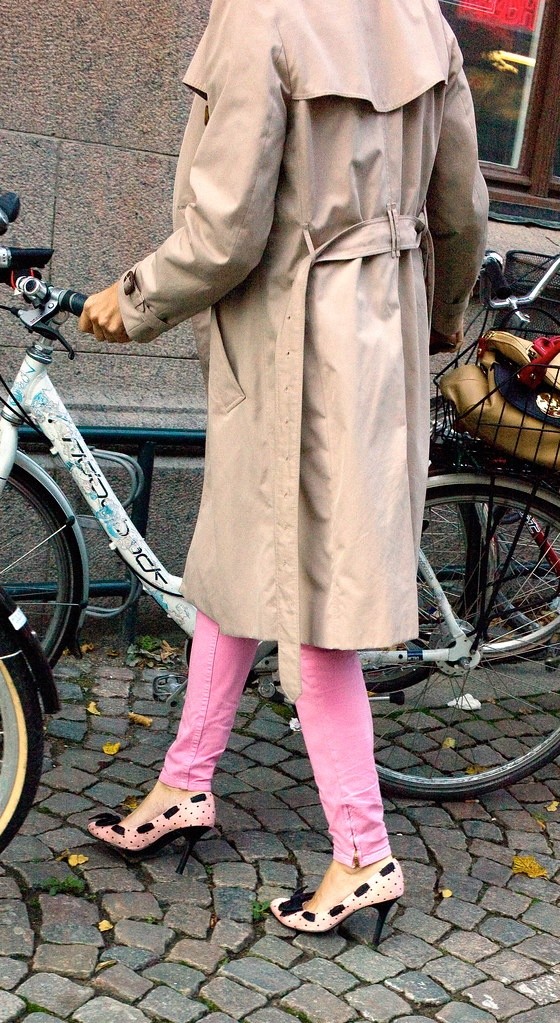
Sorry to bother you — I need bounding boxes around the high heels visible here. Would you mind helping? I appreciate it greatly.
[88,791,216,875]
[270,858,404,945]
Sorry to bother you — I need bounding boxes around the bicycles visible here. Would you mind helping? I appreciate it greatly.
[0,191,560,855]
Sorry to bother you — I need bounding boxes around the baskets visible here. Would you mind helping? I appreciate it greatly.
[493,249,560,340]
[432,325,560,480]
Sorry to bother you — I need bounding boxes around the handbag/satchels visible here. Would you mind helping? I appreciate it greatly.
[441,330,560,467]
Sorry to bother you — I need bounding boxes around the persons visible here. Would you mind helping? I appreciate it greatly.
[77,0,489,945]
